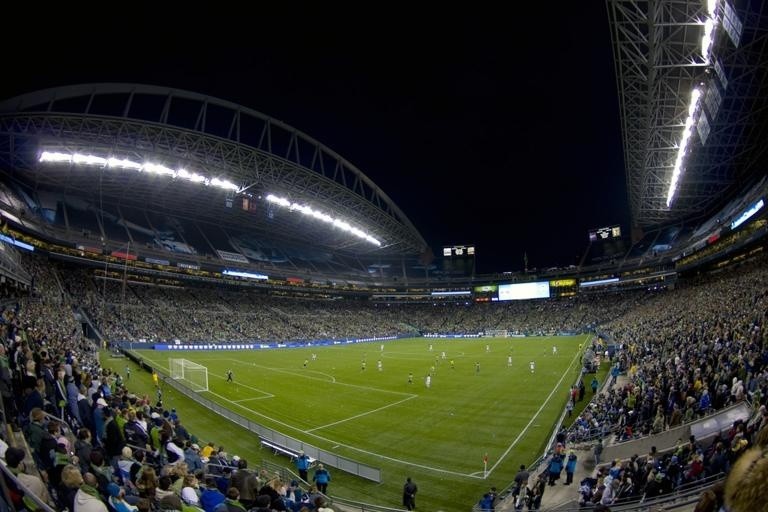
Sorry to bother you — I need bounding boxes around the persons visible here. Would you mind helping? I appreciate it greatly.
[0,239,768,511]
